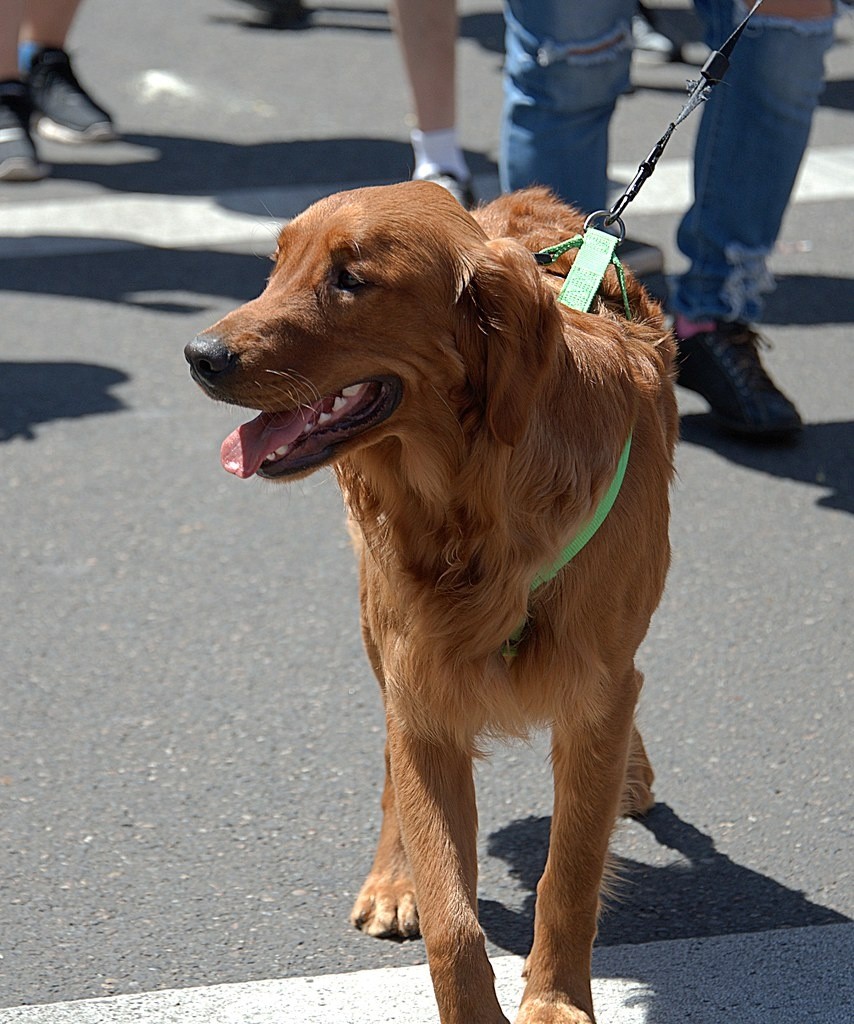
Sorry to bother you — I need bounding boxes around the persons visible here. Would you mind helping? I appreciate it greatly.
[0,0,112,181]
[383,0,476,211]
[499,0,849,450]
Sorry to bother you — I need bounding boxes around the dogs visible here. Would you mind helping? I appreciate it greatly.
[182,178,679,1024]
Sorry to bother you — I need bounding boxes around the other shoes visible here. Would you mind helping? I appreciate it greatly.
[410,154,475,217]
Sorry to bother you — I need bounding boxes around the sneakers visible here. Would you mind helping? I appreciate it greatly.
[675,320,802,443]
[31,45,115,143]
[0,81,50,181]
